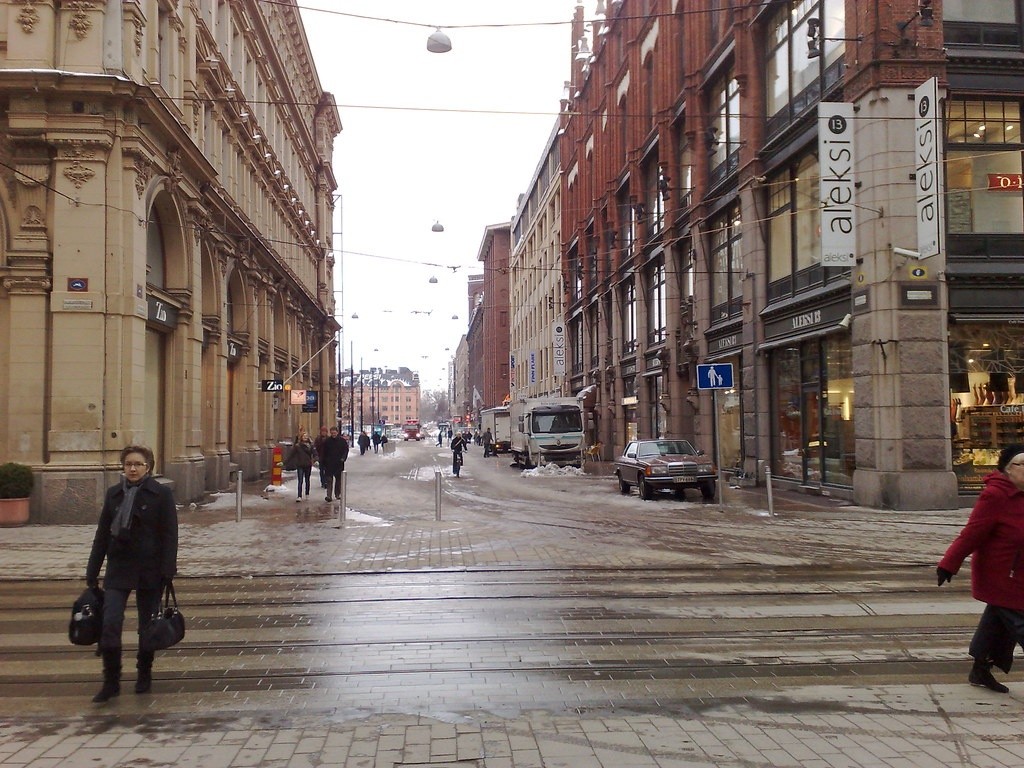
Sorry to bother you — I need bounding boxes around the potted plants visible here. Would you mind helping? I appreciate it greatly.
[0,462,35,528]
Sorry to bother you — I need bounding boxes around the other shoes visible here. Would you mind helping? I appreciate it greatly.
[325,497,332,502]
[969,663,1009,693]
[306,495,309,500]
[296,497,302,502]
[336,495,341,499]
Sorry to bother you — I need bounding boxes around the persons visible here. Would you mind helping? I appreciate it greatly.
[473,431,479,445]
[482,427,493,458]
[477,428,482,445]
[936,444,1024,693]
[451,432,467,466]
[86,443,178,702]
[371,432,380,454]
[357,431,369,455]
[314,426,329,488]
[294,427,311,447]
[448,428,453,438]
[436,431,443,447]
[462,431,472,444]
[323,426,349,502]
[290,432,319,502]
[380,434,388,448]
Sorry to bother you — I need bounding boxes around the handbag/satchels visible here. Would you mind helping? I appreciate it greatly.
[143,581,185,651]
[69,584,103,645]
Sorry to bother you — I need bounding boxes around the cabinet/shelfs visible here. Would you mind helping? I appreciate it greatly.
[962,414,1024,449]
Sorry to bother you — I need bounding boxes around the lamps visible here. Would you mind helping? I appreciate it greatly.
[452,314,458,319]
[431,220,444,233]
[838,312,855,329]
[427,26,452,53]
[547,124,748,309]
[429,275,438,283]
[352,312,358,318]
[805,0,934,59]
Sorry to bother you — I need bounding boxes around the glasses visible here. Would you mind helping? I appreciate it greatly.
[1011,462,1024,468]
[122,461,148,469]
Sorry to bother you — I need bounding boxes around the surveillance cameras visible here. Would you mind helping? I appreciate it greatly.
[893,247,919,260]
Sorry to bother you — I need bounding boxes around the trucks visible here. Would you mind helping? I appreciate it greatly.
[507,397,594,471]
[478,406,511,453]
[402,419,422,442]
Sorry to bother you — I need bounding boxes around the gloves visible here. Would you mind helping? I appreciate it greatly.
[936,566,953,586]
[314,462,319,466]
[87,574,98,586]
[161,574,174,583]
[277,462,284,467]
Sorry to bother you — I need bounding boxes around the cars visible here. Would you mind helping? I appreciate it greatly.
[613,437,719,501]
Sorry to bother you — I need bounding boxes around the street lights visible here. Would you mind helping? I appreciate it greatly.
[360,348,378,433]
[372,365,388,436]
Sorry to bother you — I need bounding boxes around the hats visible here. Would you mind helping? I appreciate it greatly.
[997,445,1024,473]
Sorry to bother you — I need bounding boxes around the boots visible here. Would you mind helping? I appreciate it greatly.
[134,663,153,692]
[93,666,122,701]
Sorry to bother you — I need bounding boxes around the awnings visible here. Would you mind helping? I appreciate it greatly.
[575,385,596,407]
